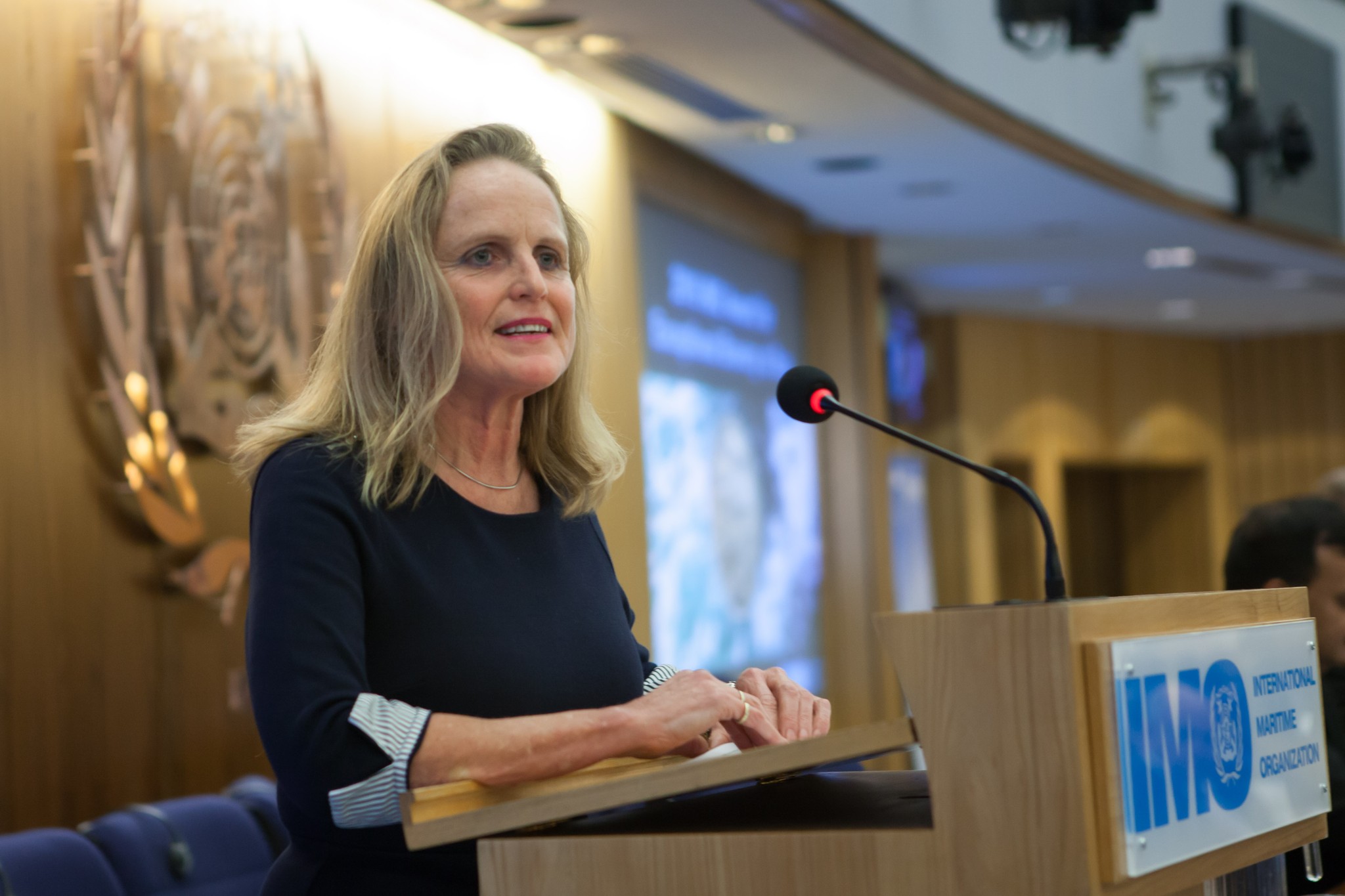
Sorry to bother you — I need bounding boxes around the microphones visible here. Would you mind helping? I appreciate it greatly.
[775,364,1070,598]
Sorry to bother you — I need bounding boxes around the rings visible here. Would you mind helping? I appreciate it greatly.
[735,702,750,725]
[738,689,745,702]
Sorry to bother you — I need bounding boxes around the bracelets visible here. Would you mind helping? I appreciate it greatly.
[703,680,737,740]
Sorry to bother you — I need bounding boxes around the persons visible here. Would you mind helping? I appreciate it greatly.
[229,116,830,896]
[1225,495,1345,896]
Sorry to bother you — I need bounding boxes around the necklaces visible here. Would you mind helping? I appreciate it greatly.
[424,435,524,490]
